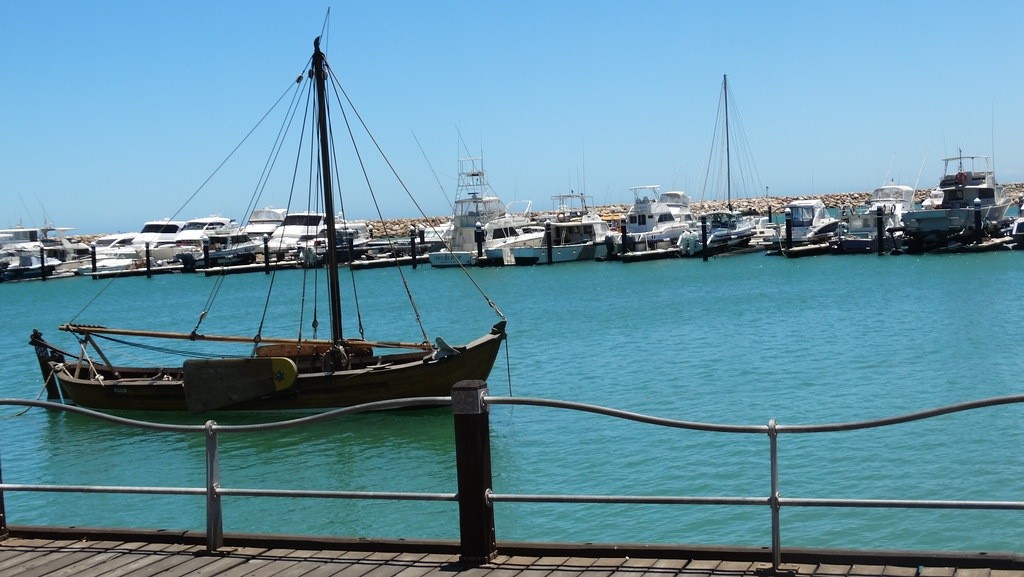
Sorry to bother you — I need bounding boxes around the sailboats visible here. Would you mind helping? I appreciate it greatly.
[32,4,506,411]
[679,72,769,257]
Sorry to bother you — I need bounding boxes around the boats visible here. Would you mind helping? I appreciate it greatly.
[0,177,693,280]
[748,149,1024,258]
